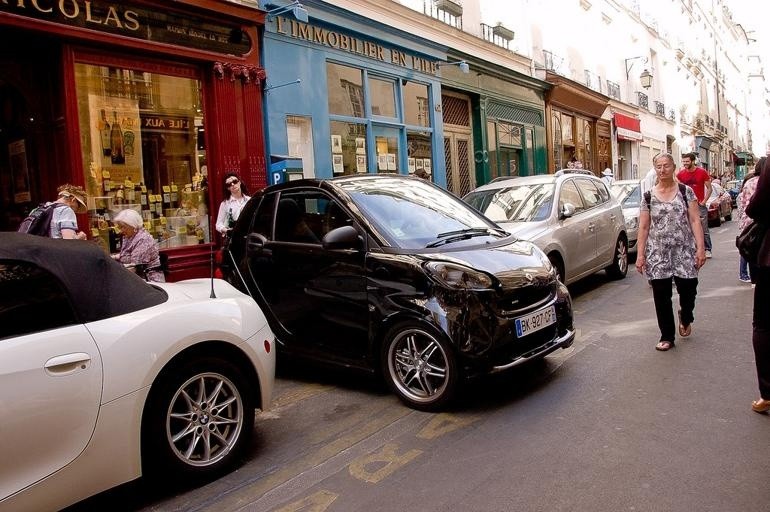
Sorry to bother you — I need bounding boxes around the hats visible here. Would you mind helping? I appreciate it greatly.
[602,168,613,176]
[60,191,86,210]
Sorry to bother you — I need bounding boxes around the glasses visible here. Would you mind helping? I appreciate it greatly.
[226,180,240,188]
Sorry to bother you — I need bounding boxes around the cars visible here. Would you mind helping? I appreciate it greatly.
[704,183,734,226]
[460,169,630,286]
[0,231,277,511]
[608,179,642,255]
[728,186,742,208]
[225,173,576,409]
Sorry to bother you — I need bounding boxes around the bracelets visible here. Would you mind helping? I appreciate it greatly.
[114,254,117,259]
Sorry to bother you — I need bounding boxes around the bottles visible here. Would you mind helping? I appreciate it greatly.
[114,224,121,251]
[160,227,169,248]
[102,167,110,191]
[109,226,117,254]
[109,111,125,165]
[143,210,151,230]
[159,213,166,232]
[93,197,111,231]
[196,225,205,244]
[170,185,177,208]
[110,176,154,211]
[176,216,187,245]
[163,188,170,208]
[155,193,162,217]
[99,109,110,157]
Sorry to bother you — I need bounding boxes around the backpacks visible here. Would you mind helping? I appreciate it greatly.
[16,202,72,235]
[643,184,690,211]
[603,177,613,189]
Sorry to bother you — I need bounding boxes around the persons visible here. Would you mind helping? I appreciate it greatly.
[742,157,767,288]
[110,209,165,283]
[44,184,88,240]
[736,174,755,284]
[676,153,713,258]
[747,155,770,412]
[413,168,430,179]
[215,173,252,243]
[711,176,720,185]
[721,172,729,182]
[636,153,706,351]
[600,168,615,192]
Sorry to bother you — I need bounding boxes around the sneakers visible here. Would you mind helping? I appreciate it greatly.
[705,250,712,259]
[739,275,751,283]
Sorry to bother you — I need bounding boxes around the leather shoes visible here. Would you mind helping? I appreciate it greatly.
[752,397,770,412]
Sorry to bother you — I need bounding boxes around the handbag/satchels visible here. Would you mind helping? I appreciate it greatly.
[736,219,770,263]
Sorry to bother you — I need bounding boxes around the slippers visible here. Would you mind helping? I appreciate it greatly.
[678,311,692,337]
[655,340,674,351]
